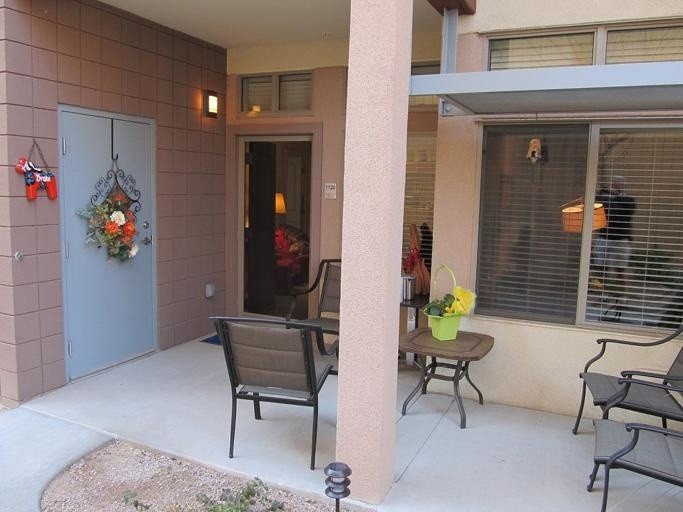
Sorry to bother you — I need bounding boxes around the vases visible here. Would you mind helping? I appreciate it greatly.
[426,314,462,341]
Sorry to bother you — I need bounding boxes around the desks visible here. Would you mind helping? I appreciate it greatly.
[398,326,495,430]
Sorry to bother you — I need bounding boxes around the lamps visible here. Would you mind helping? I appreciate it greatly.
[560,196,609,235]
[201,89,219,118]
[524,112,542,163]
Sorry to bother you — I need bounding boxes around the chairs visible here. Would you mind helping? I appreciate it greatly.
[572,315,683,512]
[275,223,309,278]
[210,257,342,470]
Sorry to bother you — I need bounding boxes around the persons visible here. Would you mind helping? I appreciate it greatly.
[594,172,636,307]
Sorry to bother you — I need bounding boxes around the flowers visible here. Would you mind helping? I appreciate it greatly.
[420,262,479,318]
[76,193,140,265]
